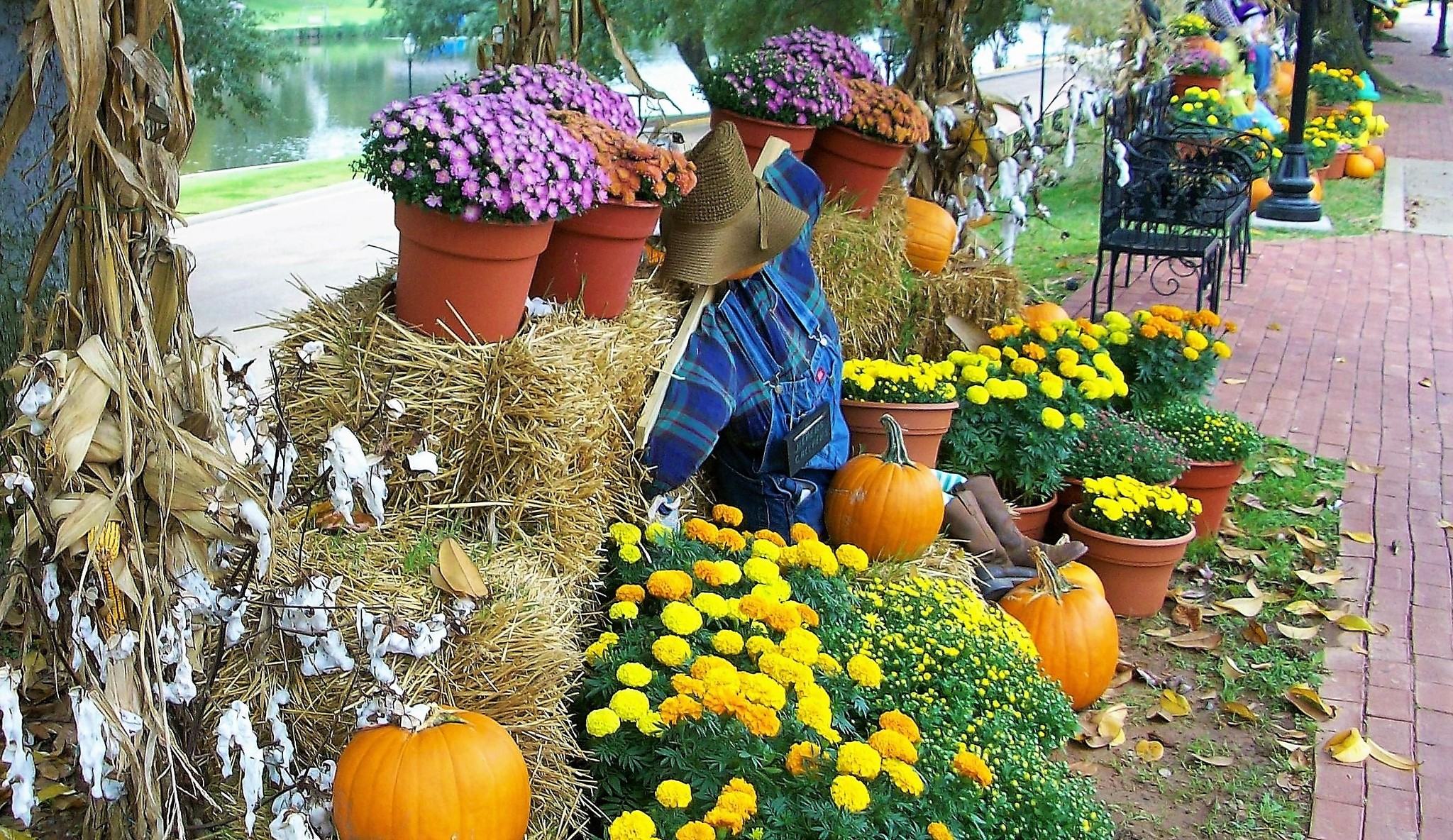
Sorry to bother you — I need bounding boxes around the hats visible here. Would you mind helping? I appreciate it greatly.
[661,121,809,285]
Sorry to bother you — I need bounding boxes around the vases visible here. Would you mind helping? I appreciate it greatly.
[1177,138,1220,159]
[1375,23,1384,30]
[710,110,818,173]
[1315,151,1347,183]
[530,202,664,319]
[1174,74,1222,96]
[1046,477,1089,535]
[804,125,914,219]
[1013,492,1057,543]
[1177,459,1246,541]
[390,196,555,345]
[1064,504,1196,620]
[841,400,960,468]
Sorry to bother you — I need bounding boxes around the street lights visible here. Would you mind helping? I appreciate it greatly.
[1037,8,1052,122]
[402,33,417,99]
[879,27,910,87]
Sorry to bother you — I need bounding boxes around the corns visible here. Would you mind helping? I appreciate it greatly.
[87,519,128,636]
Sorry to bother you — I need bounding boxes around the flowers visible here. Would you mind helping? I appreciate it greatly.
[1162,13,1366,168]
[842,305,1265,539]
[353,29,927,222]
[1372,8,1384,24]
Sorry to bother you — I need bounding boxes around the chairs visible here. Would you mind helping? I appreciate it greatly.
[1090,76,1274,337]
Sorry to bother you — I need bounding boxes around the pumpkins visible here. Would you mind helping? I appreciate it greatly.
[995,546,1119,711]
[1307,172,1322,202]
[902,196,957,274]
[723,260,767,278]
[1344,153,1375,178]
[1383,20,1392,28]
[1249,173,1274,212]
[1364,144,1385,170]
[1052,533,1105,597]
[331,704,530,840]
[826,414,944,563]
[1269,60,1294,97]
